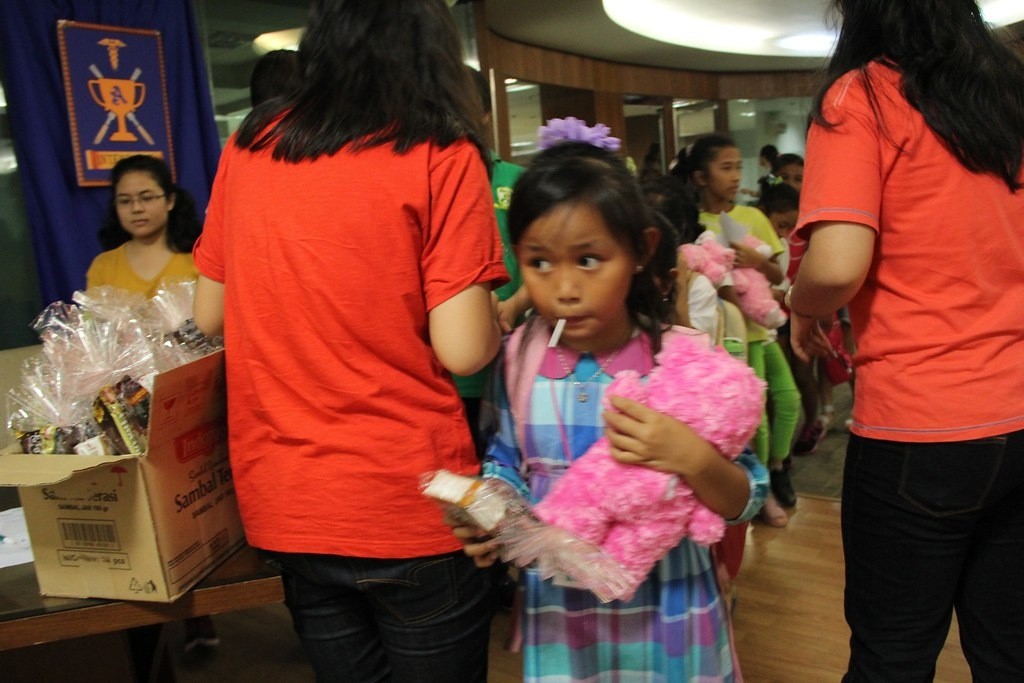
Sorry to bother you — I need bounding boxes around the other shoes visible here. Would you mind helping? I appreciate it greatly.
[819,408,838,430]
[770,468,796,508]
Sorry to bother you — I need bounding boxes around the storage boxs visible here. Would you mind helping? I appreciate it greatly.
[0,344,247,607]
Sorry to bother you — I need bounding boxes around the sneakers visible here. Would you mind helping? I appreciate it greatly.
[790,419,825,455]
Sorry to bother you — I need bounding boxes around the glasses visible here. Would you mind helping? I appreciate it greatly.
[114,192,167,206]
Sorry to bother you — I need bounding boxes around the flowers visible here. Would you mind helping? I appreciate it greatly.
[536,116,622,153]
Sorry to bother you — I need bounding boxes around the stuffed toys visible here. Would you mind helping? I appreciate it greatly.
[504,327,767,605]
[677,229,786,329]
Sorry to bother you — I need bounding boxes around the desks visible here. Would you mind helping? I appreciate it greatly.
[0,542,290,683]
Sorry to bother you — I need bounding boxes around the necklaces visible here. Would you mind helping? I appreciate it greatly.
[553,334,627,402]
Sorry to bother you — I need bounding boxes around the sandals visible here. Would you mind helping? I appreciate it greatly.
[759,502,788,527]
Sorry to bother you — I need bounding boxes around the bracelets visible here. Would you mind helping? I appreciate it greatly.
[782,281,813,320]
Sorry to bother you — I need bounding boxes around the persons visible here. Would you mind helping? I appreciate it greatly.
[787,0,1024,683]
[88,154,222,658]
[237,40,852,683]
[195,0,511,683]
